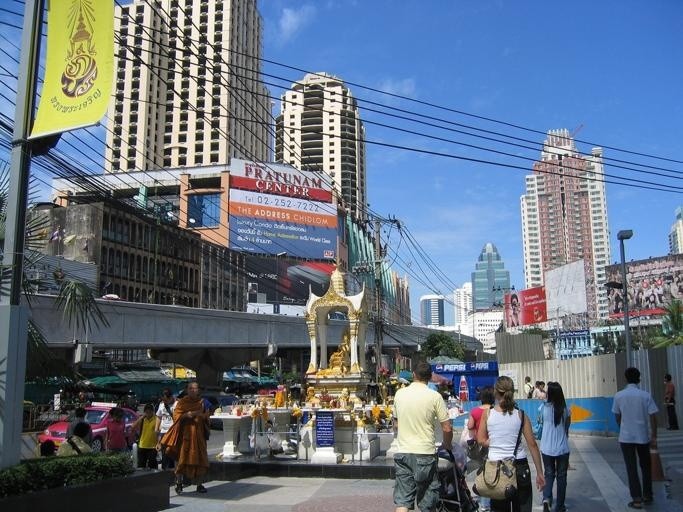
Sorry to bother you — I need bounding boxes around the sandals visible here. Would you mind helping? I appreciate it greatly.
[642,498,652,504]
[628,501,641,508]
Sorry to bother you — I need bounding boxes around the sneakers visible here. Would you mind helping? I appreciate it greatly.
[555,505,568,512]
[175,481,184,495]
[197,486,207,493]
[543,500,552,512]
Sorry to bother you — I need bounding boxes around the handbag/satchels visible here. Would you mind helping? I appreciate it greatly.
[466,438,488,460]
[533,424,543,440]
[650,450,665,481]
[473,458,518,500]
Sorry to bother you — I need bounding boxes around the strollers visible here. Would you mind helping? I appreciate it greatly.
[432,447,478,511]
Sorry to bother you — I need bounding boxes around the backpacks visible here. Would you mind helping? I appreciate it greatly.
[528,384,534,398]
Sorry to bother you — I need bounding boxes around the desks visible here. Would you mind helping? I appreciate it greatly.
[208,402,469,462]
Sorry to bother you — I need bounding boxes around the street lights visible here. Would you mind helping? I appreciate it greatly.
[605,229,634,394]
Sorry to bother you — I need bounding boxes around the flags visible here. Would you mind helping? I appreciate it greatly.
[50,231,58,240]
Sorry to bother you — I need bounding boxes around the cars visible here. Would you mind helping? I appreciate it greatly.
[39,401,143,452]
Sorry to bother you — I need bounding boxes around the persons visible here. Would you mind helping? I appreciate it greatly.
[467,387,496,512]
[132,404,161,470]
[509,294,520,327]
[663,373,679,431]
[155,388,180,469]
[65,406,87,439]
[531,380,547,399]
[522,376,534,398]
[536,382,571,512]
[604,255,683,316]
[610,366,659,510]
[473,376,547,511]
[161,382,211,494]
[365,374,410,404]
[434,378,459,402]
[391,361,453,512]
[106,407,128,452]
[54,420,93,456]
[40,439,56,455]
